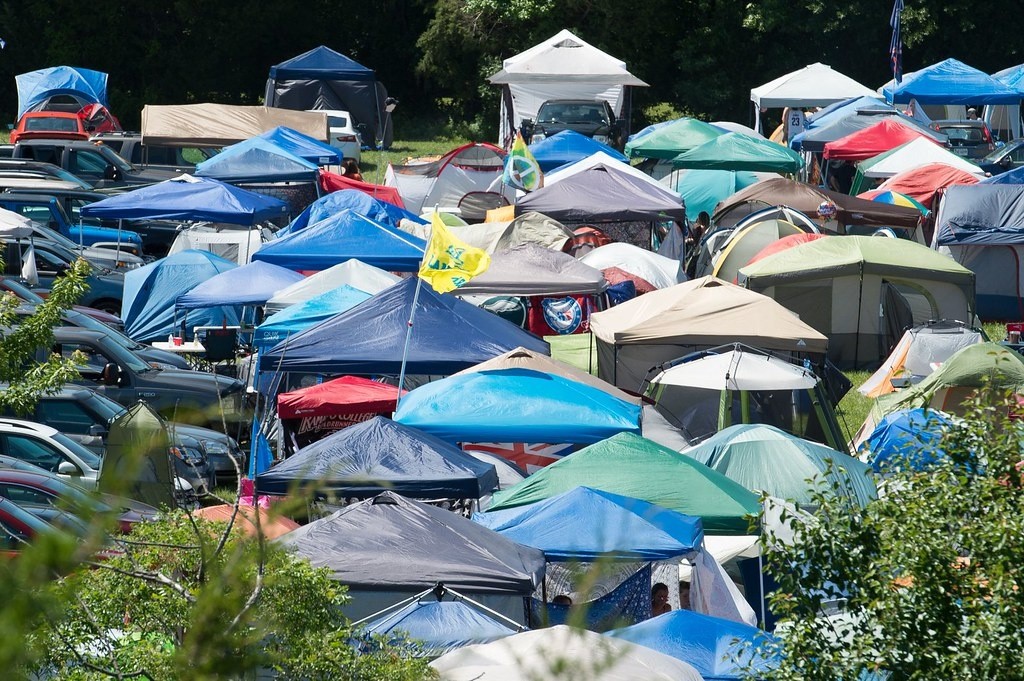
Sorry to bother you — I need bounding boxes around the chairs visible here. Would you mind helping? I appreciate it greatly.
[215,365,238,380]
[187,328,237,375]
[1007,323,1024,333]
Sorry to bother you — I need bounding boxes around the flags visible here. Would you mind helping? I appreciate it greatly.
[890,0,904,82]
[418,213,491,293]
[501,131,544,192]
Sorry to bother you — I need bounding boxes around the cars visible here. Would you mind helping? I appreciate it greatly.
[928,119,995,160]
[0,381,248,482]
[1,112,222,371]
[980,138,1024,173]
[0,326,249,440]
[304,110,367,167]
[526,98,628,151]
[0,453,177,571]
[0,388,218,502]
[0,416,200,520]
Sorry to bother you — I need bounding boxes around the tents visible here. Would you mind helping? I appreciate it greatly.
[488,28,650,151]
[0,117,1024,681]
[140,104,331,169]
[265,45,392,149]
[879,58,1024,160]
[15,66,117,131]
[751,63,886,132]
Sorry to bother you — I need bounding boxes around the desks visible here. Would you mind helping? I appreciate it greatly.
[1001,340,1024,350]
[151,342,206,371]
[193,326,242,346]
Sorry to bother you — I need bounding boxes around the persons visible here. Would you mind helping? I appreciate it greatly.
[783,107,802,143]
[967,108,982,121]
[651,582,671,617]
[342,161,363,181]
[678,581,691,610]
[694,211,709,236]
[552,596,572,605]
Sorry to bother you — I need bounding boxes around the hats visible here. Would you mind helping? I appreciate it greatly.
[967,108,976,117]
